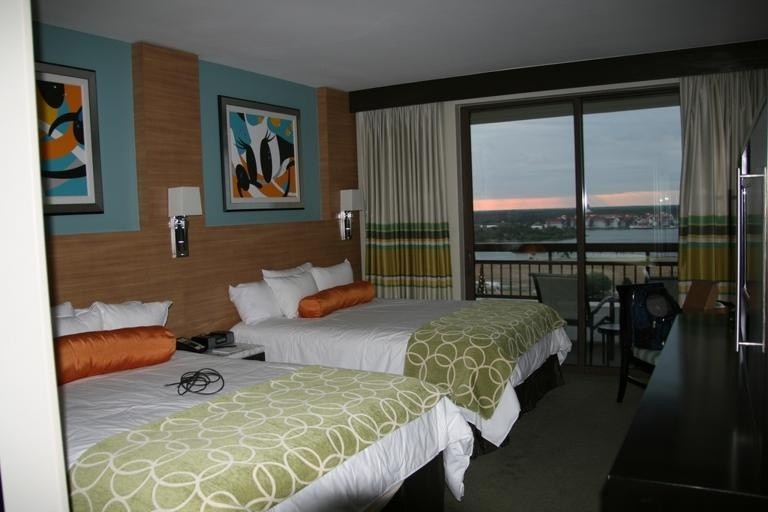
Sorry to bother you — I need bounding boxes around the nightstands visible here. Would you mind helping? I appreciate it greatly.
[208,339,268,361]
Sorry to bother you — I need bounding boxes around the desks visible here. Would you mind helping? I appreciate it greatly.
[597,322,623,367]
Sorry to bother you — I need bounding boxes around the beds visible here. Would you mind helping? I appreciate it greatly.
[47,300,476,510]
[224,259,574,448]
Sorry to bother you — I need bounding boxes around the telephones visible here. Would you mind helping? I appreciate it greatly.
[176,337,207,353]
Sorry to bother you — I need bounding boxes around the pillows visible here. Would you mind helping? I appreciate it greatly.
[51,293,177,384]
[227,256,377,326]
[627,290,679,350]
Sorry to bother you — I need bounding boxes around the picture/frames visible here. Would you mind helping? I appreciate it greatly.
[35,58,105,217]
[216,92,307,214]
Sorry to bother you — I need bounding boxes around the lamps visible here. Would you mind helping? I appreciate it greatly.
[166,185,204,260]
[337,186,365,242]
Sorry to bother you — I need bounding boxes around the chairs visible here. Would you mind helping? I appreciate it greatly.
[613,272,679,407]
[531,271,613,371]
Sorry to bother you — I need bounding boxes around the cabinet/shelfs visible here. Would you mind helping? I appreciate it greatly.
[681,277,718,311]
[596,302,766,510]
